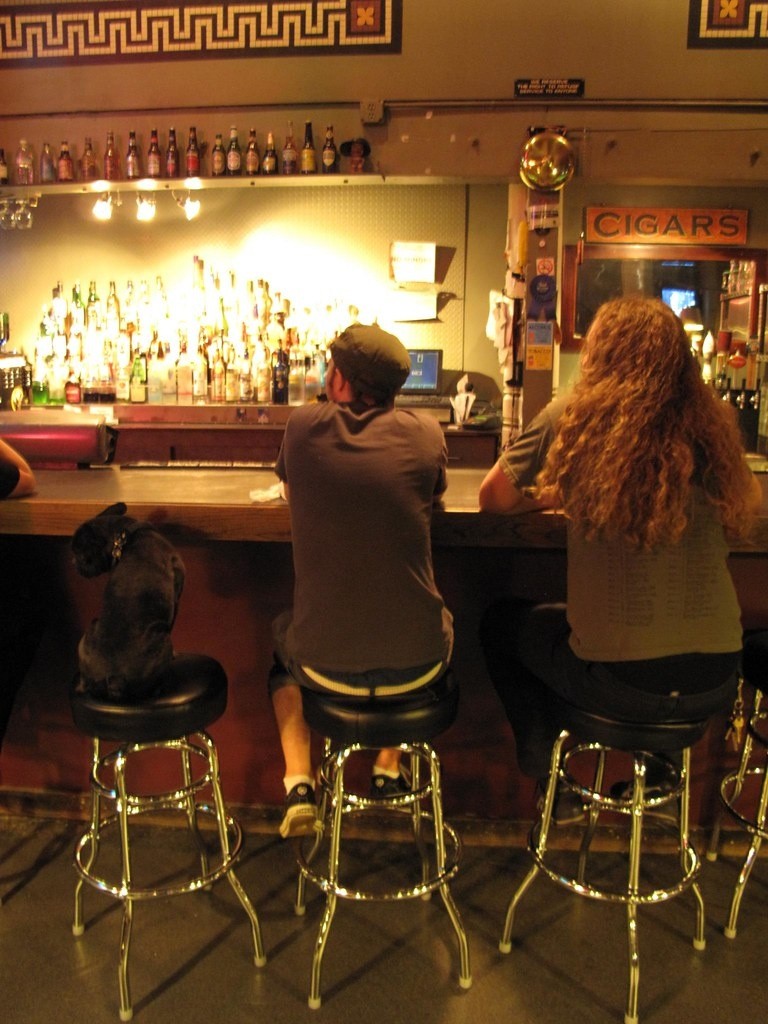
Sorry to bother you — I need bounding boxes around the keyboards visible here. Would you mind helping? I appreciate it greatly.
[394,393,452,409]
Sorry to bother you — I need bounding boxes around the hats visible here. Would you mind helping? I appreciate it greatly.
[328,323,411,397]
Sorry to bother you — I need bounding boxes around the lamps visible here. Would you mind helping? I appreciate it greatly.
[131,179,159,222]
[171,176,208,220]
[90,178,114,221]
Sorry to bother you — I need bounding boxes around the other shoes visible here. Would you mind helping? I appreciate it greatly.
[533,767,584,826]
[609,767,678,802]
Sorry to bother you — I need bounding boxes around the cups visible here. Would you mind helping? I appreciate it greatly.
[453,410,469,425]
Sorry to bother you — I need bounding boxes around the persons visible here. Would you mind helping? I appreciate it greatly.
[269,324,454,838]
[0,439,37,499]
[479,295,764,825]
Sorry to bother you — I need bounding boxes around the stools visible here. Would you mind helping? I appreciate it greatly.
[69,656,266,1021]
[290,673,473,1010]
[499,706,707,1024]
[704,613,768,938]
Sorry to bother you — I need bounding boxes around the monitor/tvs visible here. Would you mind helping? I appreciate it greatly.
[397,350,442,395]
[662,288,696,318]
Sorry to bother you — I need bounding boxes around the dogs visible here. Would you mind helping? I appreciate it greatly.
[70,502,187,695]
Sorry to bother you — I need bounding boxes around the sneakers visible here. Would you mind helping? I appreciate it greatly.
[279,784,318,839]
[369,773,413,799]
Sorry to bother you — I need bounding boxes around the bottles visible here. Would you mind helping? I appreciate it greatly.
[30,256,379,406]
[58,140,73,183]
[0,149,8,186]
[81,137,96,181]
[322,124,337,174]
[40,143,54,185]
[282,120,298,175]
[166,128,180,178]
[212,134,226,177]
[185,127,200,177]
[15,139,33,185]
[301,119,317,175]
[148,128,161,178]
[246,128,260,176]
[104,129,117,181]
[262,132,278,176]
[226,125,242,176]
[126,130,139,179]
